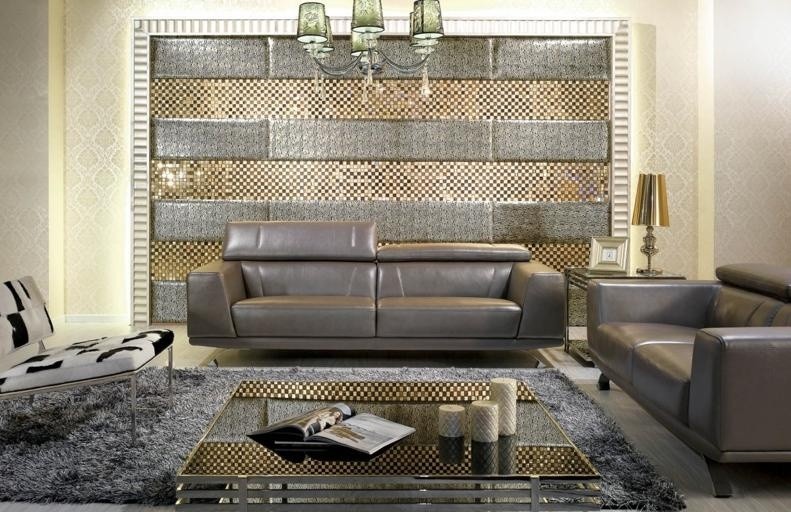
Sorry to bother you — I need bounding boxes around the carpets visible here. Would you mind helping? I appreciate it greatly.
[2,363,685,512]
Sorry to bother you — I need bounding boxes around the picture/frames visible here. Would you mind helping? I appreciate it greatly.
[588,235,632,276]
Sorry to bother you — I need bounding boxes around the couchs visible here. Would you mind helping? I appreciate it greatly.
[585,261,791,497]
[185,219,567,351]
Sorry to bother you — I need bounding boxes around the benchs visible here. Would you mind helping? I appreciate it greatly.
[0,275,176,443]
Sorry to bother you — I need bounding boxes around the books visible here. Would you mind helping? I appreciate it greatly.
[244,402,416,456]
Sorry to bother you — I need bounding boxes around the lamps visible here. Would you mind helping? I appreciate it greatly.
[632,172,672,275]
[295,0,446,103]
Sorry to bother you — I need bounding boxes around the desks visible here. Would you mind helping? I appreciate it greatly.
[565,265,685,368]
